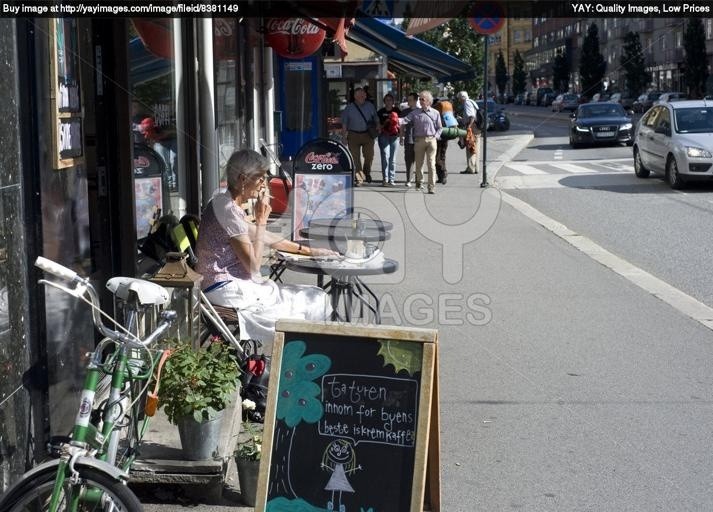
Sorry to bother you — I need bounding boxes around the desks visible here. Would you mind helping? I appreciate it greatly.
[275,216,398,323]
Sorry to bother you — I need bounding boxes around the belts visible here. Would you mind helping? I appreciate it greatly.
[353,130,366,134]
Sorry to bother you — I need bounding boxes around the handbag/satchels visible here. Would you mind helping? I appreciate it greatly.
[367,119,378,138]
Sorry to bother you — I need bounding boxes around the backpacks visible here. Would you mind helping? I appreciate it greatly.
[468,100,489,131]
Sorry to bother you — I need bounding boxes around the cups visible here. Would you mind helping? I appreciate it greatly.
[346,240,366,257]
[350,221,366,236]
[365,244,373,257]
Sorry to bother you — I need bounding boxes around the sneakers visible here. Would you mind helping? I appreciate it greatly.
[356,175,446,194]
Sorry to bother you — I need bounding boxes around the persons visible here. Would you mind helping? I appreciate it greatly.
[389,90,442,194]
[341,84,380,187]
[399,93,421,186]
[377,94,401,187]
[432,96,458,184]
[196,150,339,351]
[456,90,478,173]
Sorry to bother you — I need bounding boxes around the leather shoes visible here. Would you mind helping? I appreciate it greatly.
[461,169,477,173]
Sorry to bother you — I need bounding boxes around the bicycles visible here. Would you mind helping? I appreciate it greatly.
[0,249,176,511]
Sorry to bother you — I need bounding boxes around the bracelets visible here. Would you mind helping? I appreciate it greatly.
[255,223,266,226]
[296,242,301,254]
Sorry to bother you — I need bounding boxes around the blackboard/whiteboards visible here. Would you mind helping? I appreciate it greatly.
[254,320,436,511]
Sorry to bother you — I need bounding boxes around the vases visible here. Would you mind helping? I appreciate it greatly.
[235,458,259,507]
[175,412,221,461]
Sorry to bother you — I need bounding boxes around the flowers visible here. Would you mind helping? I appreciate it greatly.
[231,398,268,460]
[155,334,239,423]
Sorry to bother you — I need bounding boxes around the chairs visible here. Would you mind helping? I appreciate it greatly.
[171,215,258,355]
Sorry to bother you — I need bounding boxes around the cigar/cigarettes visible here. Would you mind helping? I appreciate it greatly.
[265,193,274,199]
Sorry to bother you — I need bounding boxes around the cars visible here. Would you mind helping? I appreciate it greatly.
[0,269,85,379]
[568,100,635,148]
[476,98,511,132]
[498,87,557,107]
[702,95,713,101]
[589,90,688,113]
[551,94,579,113]
[433,96,459,109]
[630,97,713,187]
